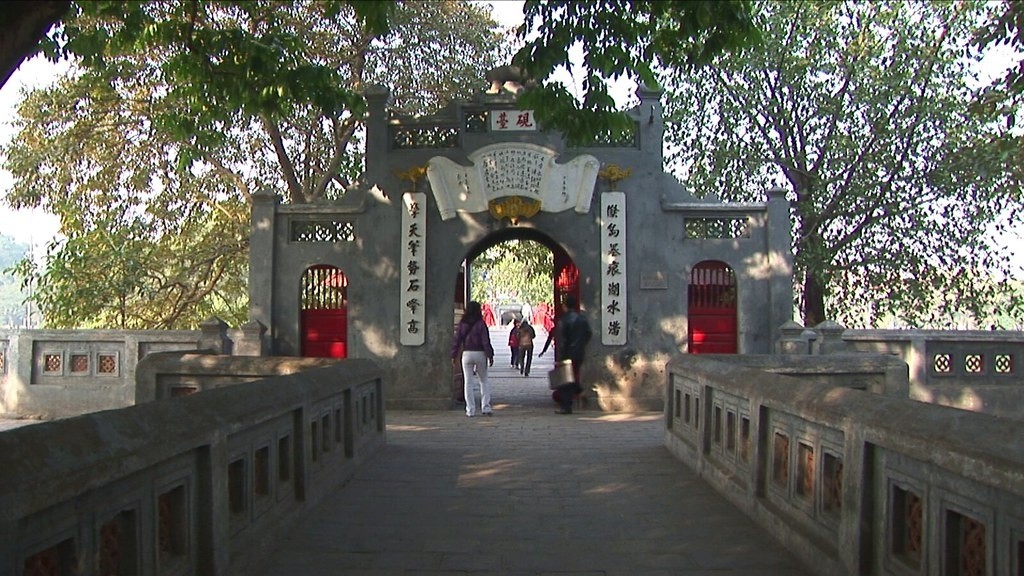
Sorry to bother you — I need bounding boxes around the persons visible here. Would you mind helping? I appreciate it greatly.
[554,292,592,415]
[449,301,495,417]
[538,315,555,357]
[514,315,536,377]
[507,318,521,369]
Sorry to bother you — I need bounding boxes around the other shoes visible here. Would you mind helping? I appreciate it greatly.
[525,374,528,376]
[482,412,494,415]
[515,366,519,369]
[521,366,524,374]
[511,366,514,368]
[555,409,573,414]
[465,413,475,417]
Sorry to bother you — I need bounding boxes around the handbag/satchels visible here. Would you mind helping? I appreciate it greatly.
[549,364,575,389]
